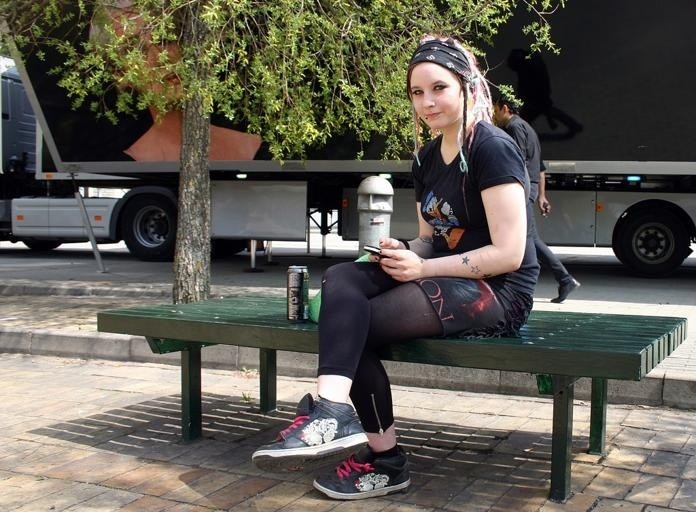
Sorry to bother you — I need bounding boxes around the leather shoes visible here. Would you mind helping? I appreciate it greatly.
[549,275,583,306]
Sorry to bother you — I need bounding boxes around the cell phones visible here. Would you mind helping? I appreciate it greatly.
[361,244,383,257]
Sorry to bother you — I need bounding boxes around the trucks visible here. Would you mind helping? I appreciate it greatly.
[0,1,696,275]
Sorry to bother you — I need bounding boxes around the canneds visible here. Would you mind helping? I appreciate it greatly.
[287,266,310,324]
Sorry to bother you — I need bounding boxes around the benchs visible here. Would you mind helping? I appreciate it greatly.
[96,290,687,506]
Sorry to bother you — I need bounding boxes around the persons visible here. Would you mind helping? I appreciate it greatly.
[251,38,540,499]
[494,95,580,302]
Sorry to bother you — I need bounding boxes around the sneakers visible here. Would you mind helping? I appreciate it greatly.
[310,441,413,502]
[250,391,371,475]
